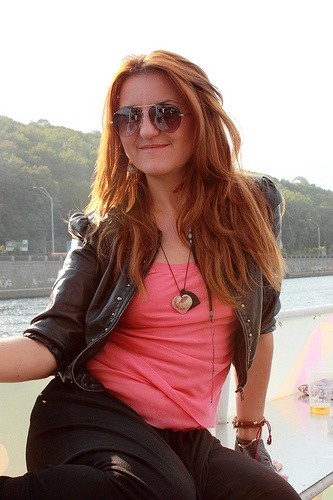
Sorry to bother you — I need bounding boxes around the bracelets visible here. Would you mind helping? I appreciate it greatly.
[238,437,257,448]
[232,414,273,459]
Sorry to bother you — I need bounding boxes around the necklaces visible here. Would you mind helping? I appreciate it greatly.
[160,241,202,314]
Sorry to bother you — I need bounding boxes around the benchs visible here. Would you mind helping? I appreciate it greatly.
[52,252,67,257]
[0,377,333,500]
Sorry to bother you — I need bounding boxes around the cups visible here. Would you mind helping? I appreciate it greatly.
[307,369,333,414]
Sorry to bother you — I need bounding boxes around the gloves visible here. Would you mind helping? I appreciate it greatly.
[234,437,277,471]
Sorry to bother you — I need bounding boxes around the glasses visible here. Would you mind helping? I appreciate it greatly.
[111,104,192,137]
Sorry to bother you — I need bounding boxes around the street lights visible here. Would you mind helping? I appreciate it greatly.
[308,218,320,248]
[32,186,54,253]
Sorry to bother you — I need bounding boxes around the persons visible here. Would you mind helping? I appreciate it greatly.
[0,50,301,500]
